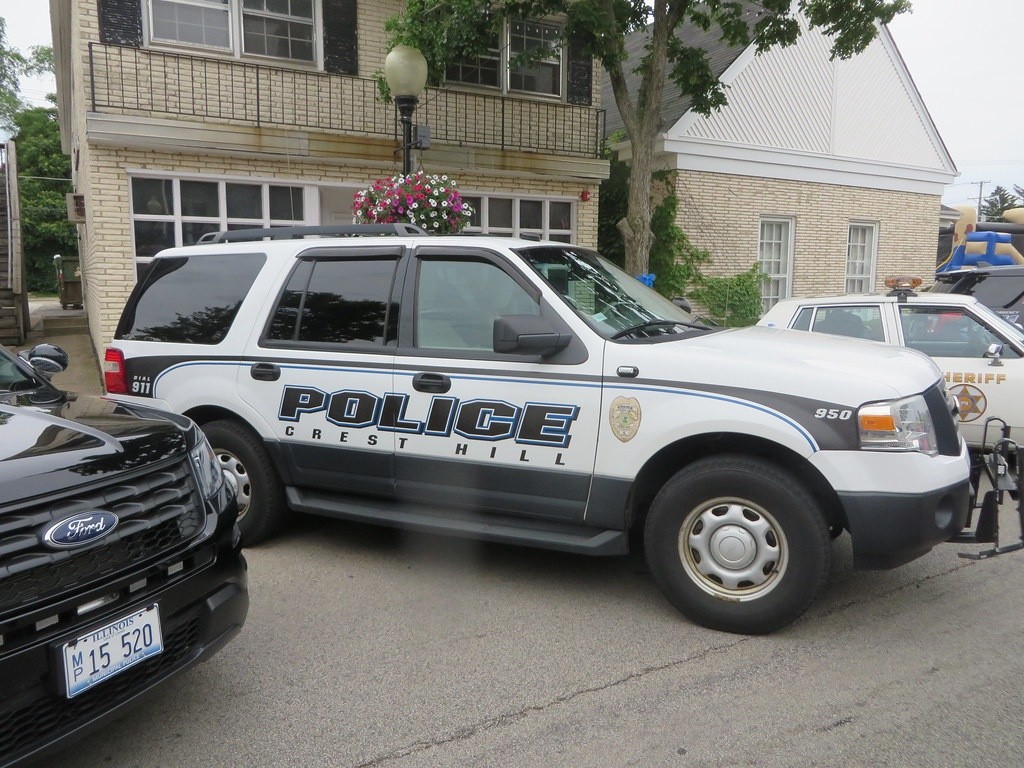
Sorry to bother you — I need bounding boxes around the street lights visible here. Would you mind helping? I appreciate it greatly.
[384,40,432,183]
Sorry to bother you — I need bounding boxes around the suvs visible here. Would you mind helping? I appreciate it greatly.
[100,220,975,637]
[903,265,1024,348]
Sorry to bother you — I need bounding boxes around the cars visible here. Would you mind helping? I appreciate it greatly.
[0,335,251,768]
[753,272,1024,456]
